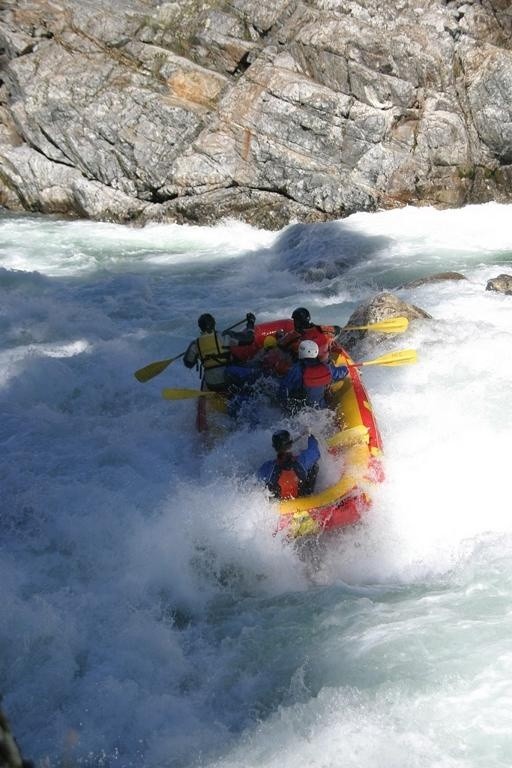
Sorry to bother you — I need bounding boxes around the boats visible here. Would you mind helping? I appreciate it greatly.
[196,321,385,539]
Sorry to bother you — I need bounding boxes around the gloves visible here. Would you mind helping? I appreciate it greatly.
[246,312,256,323]
[334,326,340,335]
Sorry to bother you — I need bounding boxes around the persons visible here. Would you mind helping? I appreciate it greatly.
[255,425,324,501]
[182,305,350,411]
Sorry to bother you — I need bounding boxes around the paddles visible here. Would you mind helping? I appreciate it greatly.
[162,387,229,401]
[135,319,248,383]
[340,317,408,334]
[328,426,367,450]
[346,349,417,368]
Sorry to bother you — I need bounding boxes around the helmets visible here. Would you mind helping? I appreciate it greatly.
[272,430,291,443]
[198,313,216,331]
[298,339,319,359]
[263,335,277,350]
[291,307,310,321]
